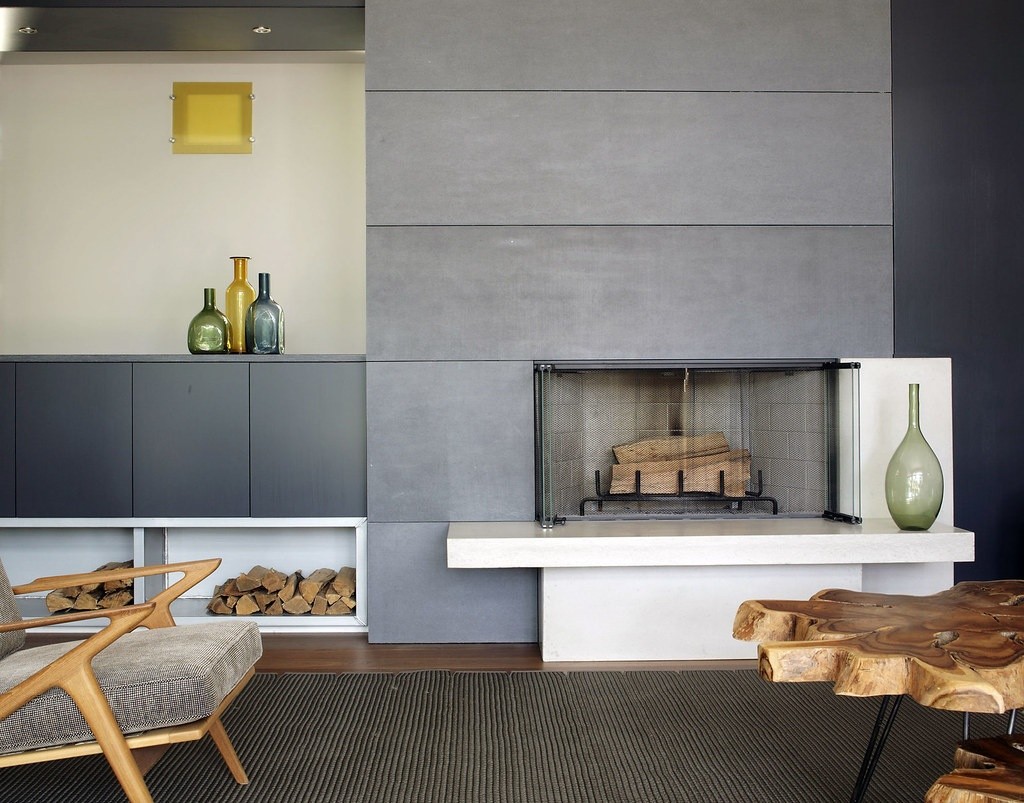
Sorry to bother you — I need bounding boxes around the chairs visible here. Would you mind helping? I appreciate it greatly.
[0,555,264,803]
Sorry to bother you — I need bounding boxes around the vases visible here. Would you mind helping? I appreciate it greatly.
[884,383,945,531]
[245,272,286,355]
[225,257,255,354]
[187,288,233,355]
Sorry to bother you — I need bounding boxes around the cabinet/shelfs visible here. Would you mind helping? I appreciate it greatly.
[0,353,369,634]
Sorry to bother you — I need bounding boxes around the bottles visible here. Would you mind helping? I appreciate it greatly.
[188,288,233,354]
[246,273,286,356]
[226,255,255,353]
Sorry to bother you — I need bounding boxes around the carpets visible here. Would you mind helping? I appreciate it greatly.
[0,663,1024,803]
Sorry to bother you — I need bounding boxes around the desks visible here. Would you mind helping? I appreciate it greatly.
[732,577,1024,803]
